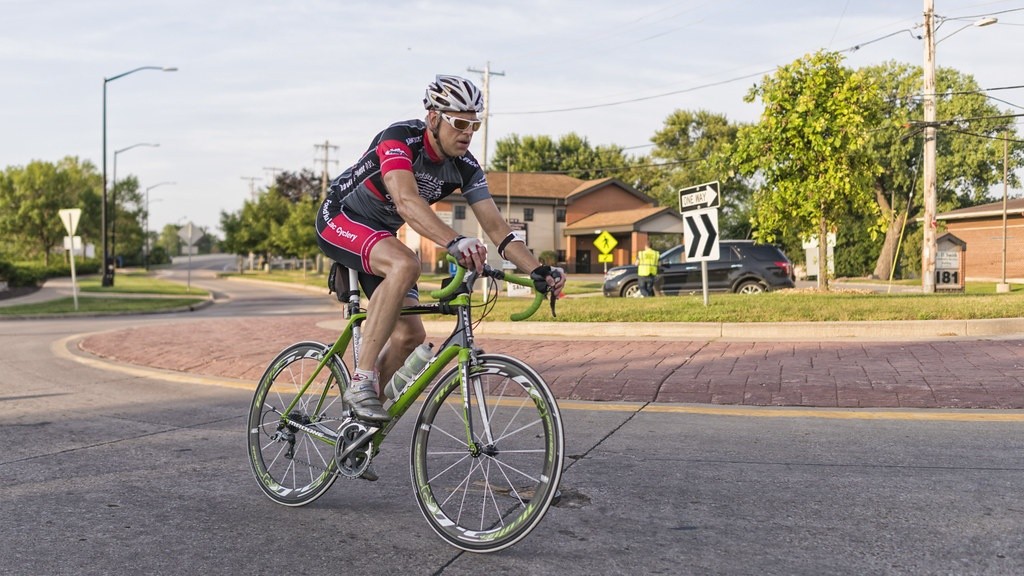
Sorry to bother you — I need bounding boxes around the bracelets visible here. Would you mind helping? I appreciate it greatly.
[497,231,524,260]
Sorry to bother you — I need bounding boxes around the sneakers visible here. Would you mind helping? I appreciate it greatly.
[342,380,390,422]
[344,452,379,482]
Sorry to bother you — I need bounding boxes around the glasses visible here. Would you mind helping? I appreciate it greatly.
[435,110,482,132]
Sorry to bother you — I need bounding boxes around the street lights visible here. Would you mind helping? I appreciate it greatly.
[994,126,1010,284]
[101,65,178,266]
[112,141,161,262]
[145,182,177,258]
[924,19,999,282]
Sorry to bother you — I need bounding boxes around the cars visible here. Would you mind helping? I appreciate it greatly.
[261,251,314,271]
[602,239,795,298]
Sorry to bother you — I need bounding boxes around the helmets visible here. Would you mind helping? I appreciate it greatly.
[423,74,484,112]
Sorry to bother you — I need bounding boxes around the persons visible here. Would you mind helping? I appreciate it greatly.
[634,241,660,297]
[315,76,567,482]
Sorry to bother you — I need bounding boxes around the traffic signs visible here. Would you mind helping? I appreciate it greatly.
[935,233,964,290]
[679,184,719,260]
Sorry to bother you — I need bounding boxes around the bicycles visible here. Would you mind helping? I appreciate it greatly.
[247,251,563,553]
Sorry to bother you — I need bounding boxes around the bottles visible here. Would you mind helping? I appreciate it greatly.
[384,342,434,400]
